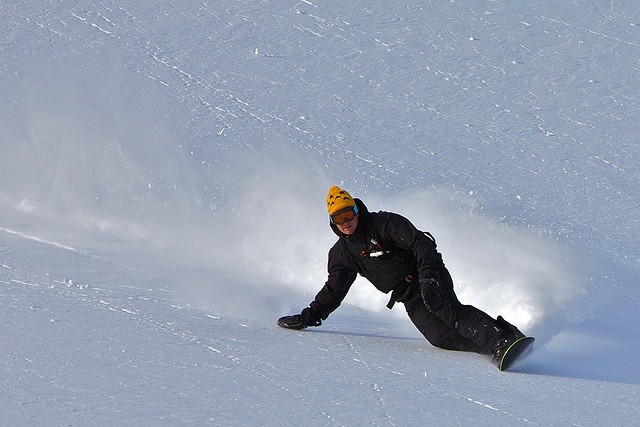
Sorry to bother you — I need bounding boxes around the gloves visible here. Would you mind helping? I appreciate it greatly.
[419,278,445,315]
[277,307,322,329]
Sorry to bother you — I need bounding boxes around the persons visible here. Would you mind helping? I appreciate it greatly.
[278,187,534,372]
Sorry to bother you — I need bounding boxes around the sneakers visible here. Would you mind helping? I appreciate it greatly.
[493,329,525,361]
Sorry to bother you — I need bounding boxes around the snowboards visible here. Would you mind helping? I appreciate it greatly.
[492,315,536,371]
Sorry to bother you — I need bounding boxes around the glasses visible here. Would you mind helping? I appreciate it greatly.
[329,205,359,224]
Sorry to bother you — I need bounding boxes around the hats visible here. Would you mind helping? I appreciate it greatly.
[326,186,355,215]
